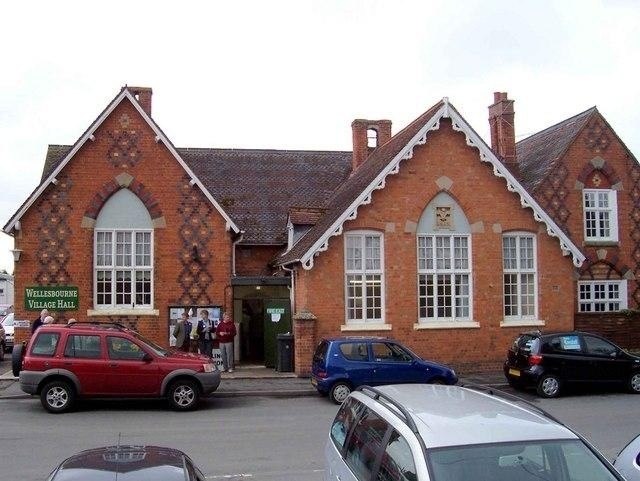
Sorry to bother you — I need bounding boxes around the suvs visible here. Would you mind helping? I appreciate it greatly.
[320,384,626,481]
[10,321,222,414]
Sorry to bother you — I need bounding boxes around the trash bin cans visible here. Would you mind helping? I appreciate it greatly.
[277,333,294,372]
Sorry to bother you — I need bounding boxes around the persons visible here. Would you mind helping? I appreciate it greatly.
[172,312,192,353]
[196,310,216,358]
[215,311,237,373]
[31,308,77,334]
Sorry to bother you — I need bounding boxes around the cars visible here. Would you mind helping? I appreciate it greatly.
[0,305,15,361]
[46,444,205,481]
[503,329,640,396]
[311,337,459,406]
[613,435,639,481]
